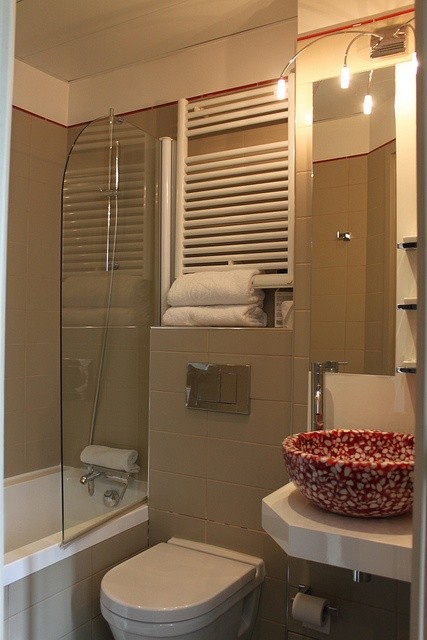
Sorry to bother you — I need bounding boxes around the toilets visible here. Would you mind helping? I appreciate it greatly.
[99,535,264,640]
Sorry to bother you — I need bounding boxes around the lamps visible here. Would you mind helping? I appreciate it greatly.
[363,68,378,116]
[274,17,383,100]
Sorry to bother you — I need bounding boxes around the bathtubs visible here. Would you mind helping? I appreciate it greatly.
[4,464,149,586]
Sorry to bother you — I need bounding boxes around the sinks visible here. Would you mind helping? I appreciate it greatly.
[282,428,416,517]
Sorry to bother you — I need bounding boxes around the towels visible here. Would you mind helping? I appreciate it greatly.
[79,444,142,473]
[168,268,265,306]
[161,305,268,326]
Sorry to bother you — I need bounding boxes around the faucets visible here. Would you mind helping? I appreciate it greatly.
[311,361,325,432]
